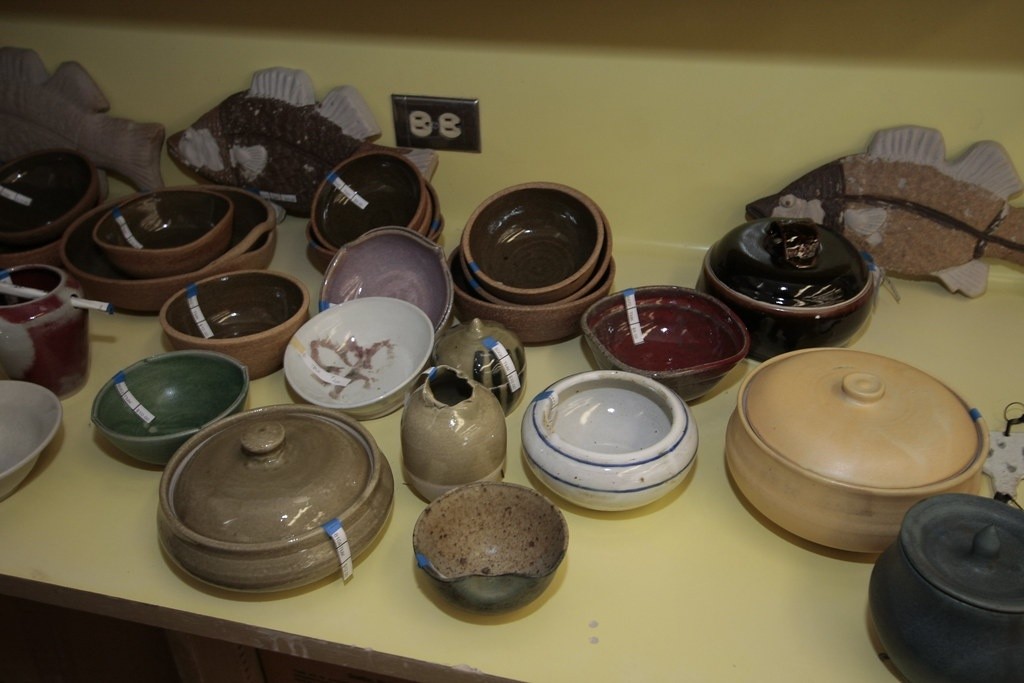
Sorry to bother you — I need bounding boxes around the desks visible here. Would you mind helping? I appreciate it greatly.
[0,239,1023,682]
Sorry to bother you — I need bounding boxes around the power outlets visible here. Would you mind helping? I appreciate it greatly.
[390,93,482,153]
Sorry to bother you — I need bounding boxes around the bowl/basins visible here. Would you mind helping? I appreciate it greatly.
[412,478,568,618]
[725,347,988,553]
[160,270,307,378]
[0,149,280,316]
[285,297,434,420]
[449,183,618,348]
[868,494,1024,683]
[705,218,878,359]
[320,227,453,343]
[582,287,747,403]
[0,381,63,499]
[158,403,394,592]
[90,350,248,466]
[526,371,698,512]
[305,152,446,274]
